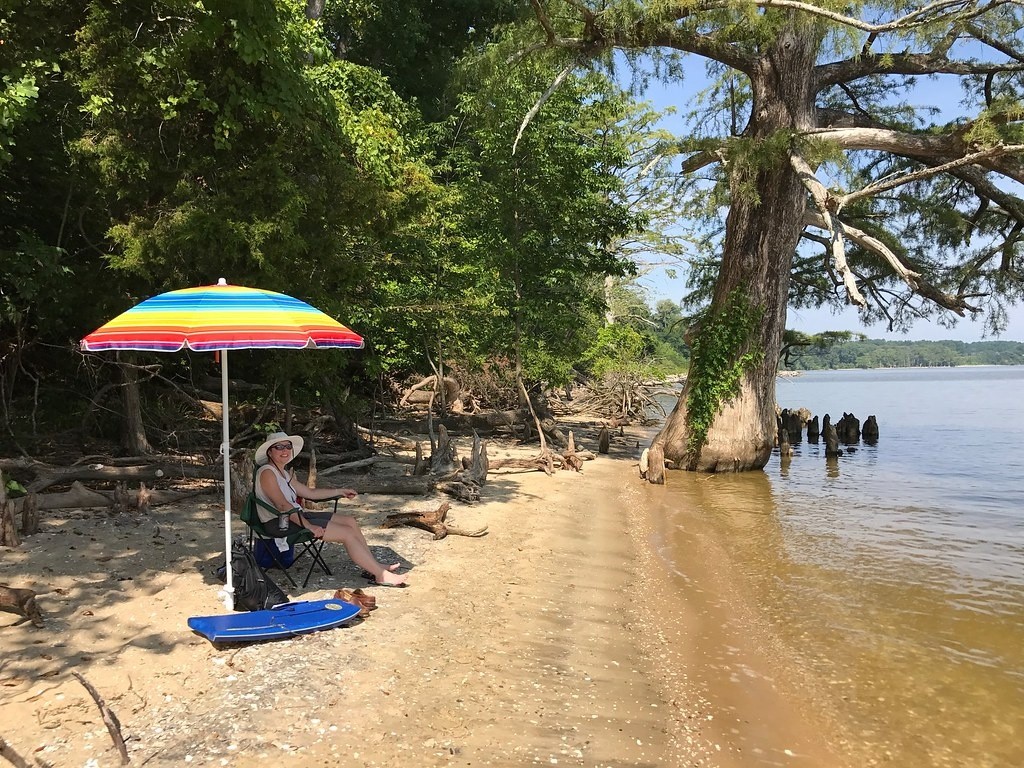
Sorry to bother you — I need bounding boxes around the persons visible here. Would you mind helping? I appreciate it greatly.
[253,432,409,587]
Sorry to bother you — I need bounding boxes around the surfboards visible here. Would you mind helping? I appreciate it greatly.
[186,598,361,644]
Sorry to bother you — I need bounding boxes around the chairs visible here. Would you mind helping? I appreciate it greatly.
[249,461,345,590]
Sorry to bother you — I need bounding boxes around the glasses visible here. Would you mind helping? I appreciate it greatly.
[271,444,292,450]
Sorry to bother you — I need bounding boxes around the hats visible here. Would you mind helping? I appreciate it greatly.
[255,432,304,466]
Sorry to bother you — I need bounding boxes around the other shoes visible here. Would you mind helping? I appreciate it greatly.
[350,588,375,610]
[333,590,369,617]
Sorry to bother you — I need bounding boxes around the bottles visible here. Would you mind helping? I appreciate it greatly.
[278,503,289,531]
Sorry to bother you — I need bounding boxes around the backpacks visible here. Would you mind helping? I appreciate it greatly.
[216,539,289,612]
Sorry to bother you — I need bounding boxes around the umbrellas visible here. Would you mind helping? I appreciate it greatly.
[79,278,366,611]
